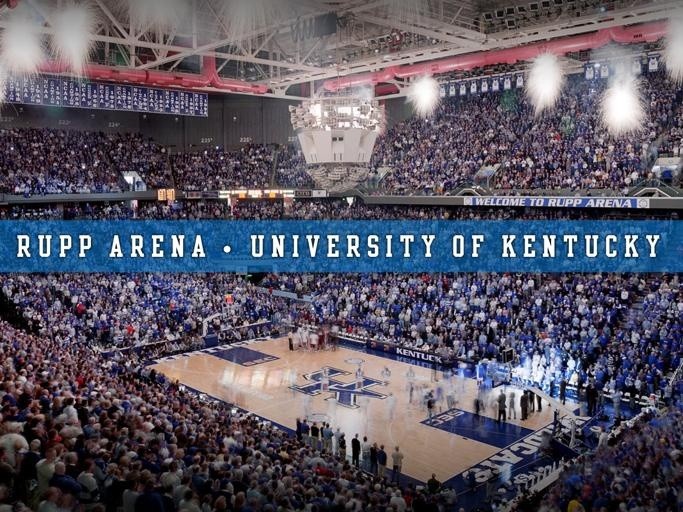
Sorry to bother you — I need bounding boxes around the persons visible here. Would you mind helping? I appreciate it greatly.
[3,69,683,512]
